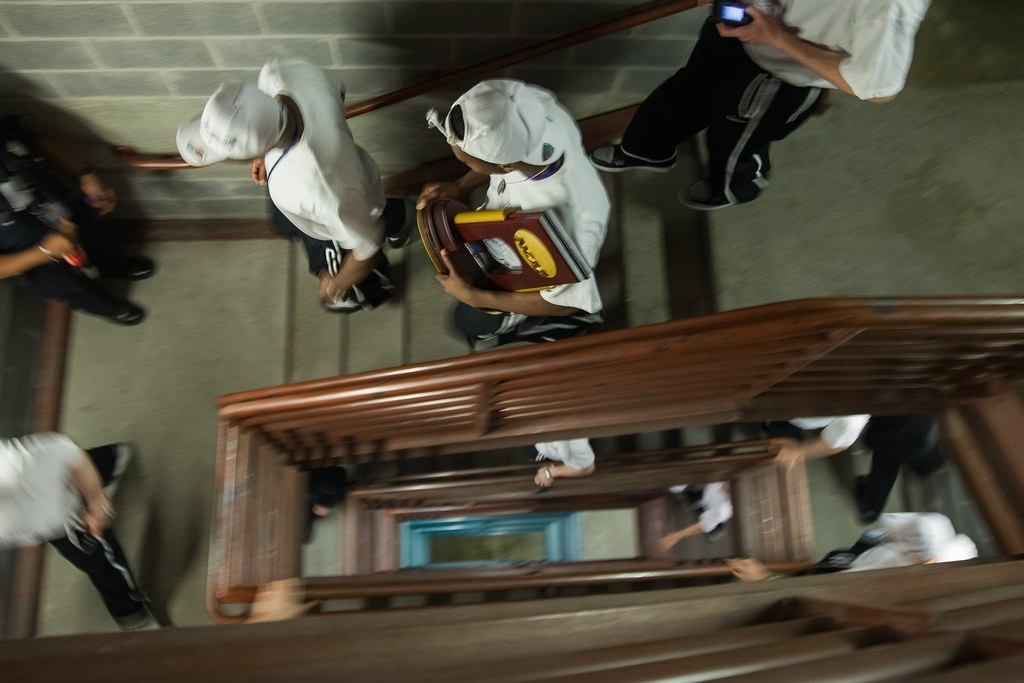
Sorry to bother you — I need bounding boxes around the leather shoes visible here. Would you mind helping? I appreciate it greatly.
[104,299,143,325]
[130,258,153,281]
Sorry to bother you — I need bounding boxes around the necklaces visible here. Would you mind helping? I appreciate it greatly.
[494,164,553,196]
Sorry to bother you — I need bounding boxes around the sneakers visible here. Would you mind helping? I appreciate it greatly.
[678,180,735,209]
[588,144,677,172]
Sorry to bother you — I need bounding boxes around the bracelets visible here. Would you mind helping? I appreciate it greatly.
[326,278,345,305]
[36,242,60,263]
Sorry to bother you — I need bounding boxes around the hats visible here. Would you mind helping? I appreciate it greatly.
[176,81,288,167]
[445,78,568,165]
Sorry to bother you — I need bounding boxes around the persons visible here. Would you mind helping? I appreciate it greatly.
[417,77,612,352]
[174,52,416,316]
[0,430,162,632]
[248,577,320,624]
[304,467,350,544]
[589,0,933,212]
[534,437,596,488]
[656,413,980,583]
[1,121,157,328]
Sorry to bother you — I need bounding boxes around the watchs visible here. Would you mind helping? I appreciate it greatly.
[544,467,554,480]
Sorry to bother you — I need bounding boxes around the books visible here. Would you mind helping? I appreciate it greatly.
[453,208,594,292]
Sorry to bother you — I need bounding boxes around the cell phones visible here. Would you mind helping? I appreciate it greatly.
[713,0,753,28]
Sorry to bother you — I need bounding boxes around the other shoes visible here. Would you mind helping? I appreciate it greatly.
[104,442,133,499]
[120,616,160,633]
[855,473,882,521]
[322,291,361,313]
